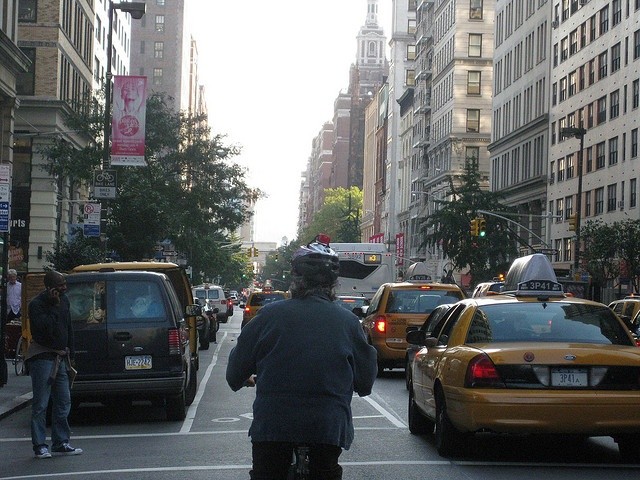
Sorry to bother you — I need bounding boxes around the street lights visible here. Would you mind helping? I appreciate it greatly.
[561,126,586,282]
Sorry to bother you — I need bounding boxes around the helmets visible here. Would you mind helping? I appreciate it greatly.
[291,233,340,283]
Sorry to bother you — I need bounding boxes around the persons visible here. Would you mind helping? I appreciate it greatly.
[6,269,22,322]
[23,265,83,459]
[113,75,148,143]
[225,232,379,480]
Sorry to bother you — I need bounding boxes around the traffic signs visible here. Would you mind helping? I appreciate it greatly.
[0,165,11,184]
[94,170,117,199]
[82,203,100,238]
[0,185,11,202]
[0,203,9,230]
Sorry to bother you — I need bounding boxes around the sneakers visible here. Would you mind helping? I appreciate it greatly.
[32,448,52,458]
[51,443,83,455]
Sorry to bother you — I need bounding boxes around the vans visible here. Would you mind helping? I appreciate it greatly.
[19,261,206,406]
[191,284,228,323]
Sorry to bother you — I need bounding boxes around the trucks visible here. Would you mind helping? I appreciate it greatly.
[327,242,396,301]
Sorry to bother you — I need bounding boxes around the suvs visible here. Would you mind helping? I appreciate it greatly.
[62,271,203,420]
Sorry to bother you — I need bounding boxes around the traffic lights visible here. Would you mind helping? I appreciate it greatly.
[470,219,478,237]
[479,219,487,238]
[568,213,575,232]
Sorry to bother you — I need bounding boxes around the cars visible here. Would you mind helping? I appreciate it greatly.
[404,303,455,389]
[405,252,639,464]
[194,297,211,350]
[198,296,220,342]
[239,281,288,330]
[334,296,369,316]
[224,291,233,316]
[608,296,640,322]
[472,282,504,298]
[352,261,467,379]
